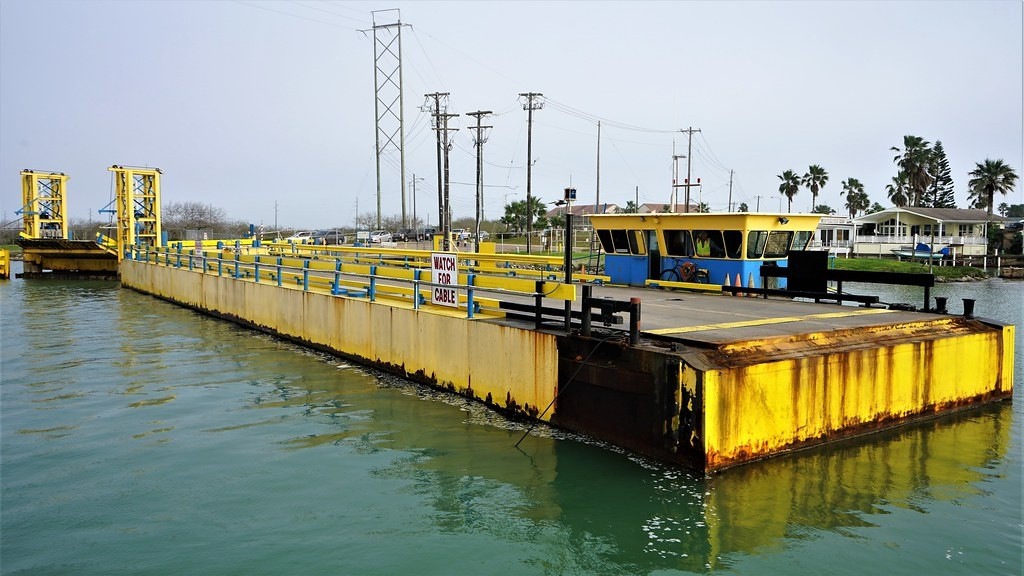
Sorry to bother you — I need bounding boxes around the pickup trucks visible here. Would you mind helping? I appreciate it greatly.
[284,232,320,245]
[452,229,472,240]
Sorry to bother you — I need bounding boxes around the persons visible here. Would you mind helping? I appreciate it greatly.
[687,230,725,259]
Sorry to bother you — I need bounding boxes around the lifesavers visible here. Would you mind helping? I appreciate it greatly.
[680,261,696,279]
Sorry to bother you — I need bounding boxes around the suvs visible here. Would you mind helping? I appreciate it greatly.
[419,229,435,240]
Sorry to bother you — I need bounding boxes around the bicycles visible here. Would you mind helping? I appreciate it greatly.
[658,257,709,292]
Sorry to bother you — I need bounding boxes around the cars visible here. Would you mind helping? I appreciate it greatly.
[308,230,345,245]
[366,230,393,244]
[392,229,420,242]
[1005,222,1024,228]
[474,231,490,240]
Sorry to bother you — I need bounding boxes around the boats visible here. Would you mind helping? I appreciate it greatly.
[581,213,830,295]
[890,248,944,259]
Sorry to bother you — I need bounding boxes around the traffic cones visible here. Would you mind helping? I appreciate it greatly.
[735,272,743,295]
[747,272,760,297]
[722,273,732,296]
